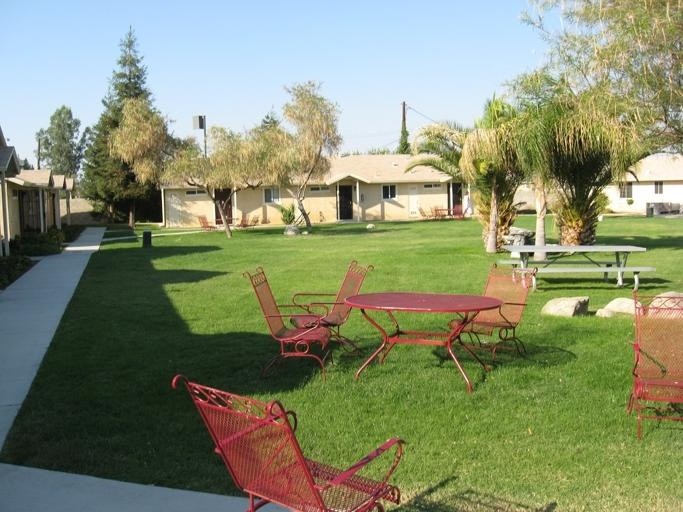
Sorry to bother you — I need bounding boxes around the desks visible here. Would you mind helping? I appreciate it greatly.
[344,288,505,394]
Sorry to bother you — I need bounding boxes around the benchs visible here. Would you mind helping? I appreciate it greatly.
[499,241,660,293]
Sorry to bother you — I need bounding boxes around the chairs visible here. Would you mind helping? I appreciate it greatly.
[243,261,337,382]
[627,284,683,439]
[197,211,259,234]
[291,257,378,364]
[170,370,413,512]
[446,261,542,371]
[414,202,471,223]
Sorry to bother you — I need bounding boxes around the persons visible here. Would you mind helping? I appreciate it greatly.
[461,191,473,218]
